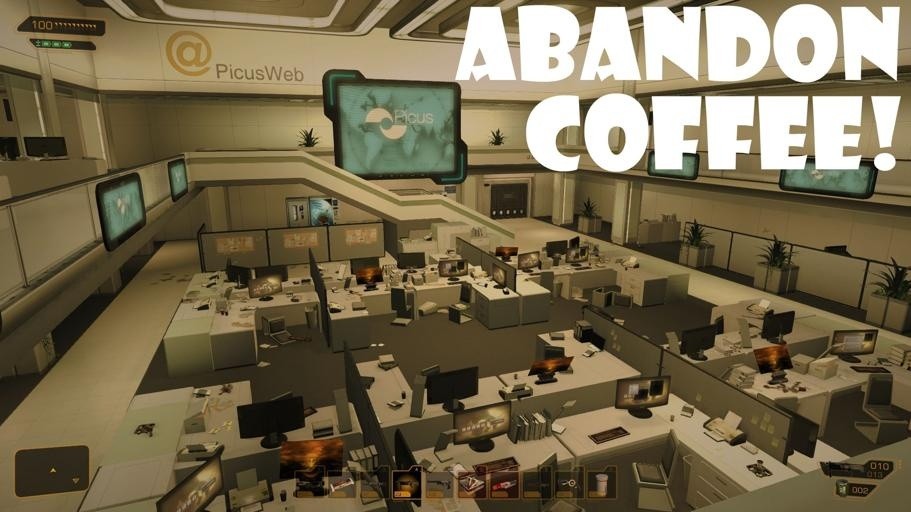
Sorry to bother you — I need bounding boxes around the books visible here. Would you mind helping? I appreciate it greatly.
[449,461,473,480]
[506,410,553,443]
[887,342,911,369]
[575,318,592,342]
[721,361,756,388]
[346,443,379,476]
[382,263,397,275]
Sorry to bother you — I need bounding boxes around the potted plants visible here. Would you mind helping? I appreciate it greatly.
[578,195,603,235]
[679,216,801,295]
